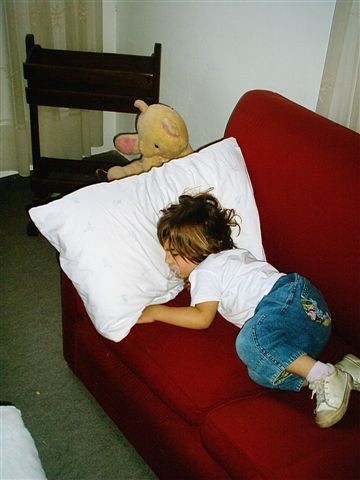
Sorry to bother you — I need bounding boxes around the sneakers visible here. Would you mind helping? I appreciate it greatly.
[309,363,354,428]
[335,354,360,392]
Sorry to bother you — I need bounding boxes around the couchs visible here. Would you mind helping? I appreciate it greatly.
[56,90,360,480]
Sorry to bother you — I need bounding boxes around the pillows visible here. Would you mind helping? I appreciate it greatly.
[29,137,266,344]
[0,406,48,480]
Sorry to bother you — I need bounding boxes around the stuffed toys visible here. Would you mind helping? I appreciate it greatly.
[107,100,193,181]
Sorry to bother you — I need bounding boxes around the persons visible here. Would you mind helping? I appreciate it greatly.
[139,186,359,428]
